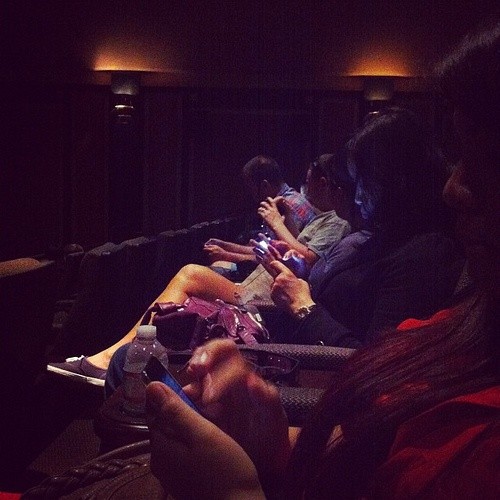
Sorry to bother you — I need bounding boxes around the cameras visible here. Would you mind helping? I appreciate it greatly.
[252,239,268,257]
[276,204,285,216]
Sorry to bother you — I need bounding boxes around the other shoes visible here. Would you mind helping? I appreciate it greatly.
[46,355,106,387]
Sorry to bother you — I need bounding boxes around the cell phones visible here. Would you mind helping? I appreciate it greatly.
[142,356,203,417]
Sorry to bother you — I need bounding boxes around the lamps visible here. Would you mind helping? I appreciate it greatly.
[364,78,393,118]
[112,82,139,127]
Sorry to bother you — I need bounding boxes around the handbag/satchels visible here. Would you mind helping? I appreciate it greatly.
[138,297,270,350]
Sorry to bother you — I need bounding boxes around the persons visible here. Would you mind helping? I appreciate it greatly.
[204,153,357,296]
[249,168,364,291]
[0,28,499,500]
[45,107,469,387]
[201,155,319,283]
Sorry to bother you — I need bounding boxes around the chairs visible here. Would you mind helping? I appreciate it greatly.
[0,216,360,500]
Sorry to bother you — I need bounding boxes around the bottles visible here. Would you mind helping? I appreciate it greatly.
[122,325,169,418]
